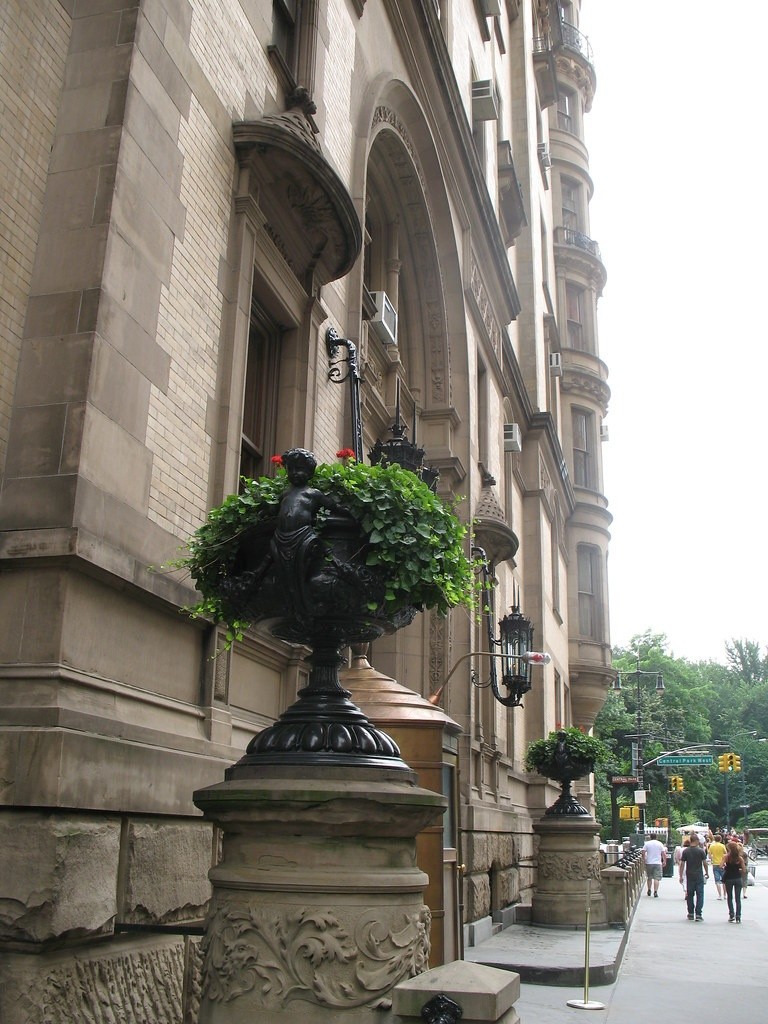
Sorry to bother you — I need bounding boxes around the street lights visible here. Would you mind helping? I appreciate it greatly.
[615,658,667,844]
[715,738,766,831]
[724,730,759,836]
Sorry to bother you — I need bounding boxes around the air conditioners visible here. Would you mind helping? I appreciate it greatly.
[369,291,399,345]
[504,423,523,453]
[600,425,610,441]
[480,0,502,17]
[550,352,562,376]
[538,142,551,167]
[472,79,501,122]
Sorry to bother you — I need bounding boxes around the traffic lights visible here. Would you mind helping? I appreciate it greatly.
[718,753,727,773]
[677,777,684,792]
[723,752,737,773]
[656,820,662,828]
[734,754,742,773]
[671,776,678,792]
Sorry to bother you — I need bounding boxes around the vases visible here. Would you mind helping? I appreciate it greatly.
[533,729,595,816]
[204,449,426,771]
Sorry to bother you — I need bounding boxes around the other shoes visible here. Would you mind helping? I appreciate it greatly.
[737,918,740,923]
[648,892,651,895]
[654,893,658,896]
[718,897,721,900]
[744,896,747,898]
[728,917,735,921]
[687,914,694,919]
[696,917,704,921]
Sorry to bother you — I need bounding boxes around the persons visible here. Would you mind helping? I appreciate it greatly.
[258,447,338,596]
[719,842,746,924]
[679,834,710,922]
[676,826,748,900]
[643,833,667,897]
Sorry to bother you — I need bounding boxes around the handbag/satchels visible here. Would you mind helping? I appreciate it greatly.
[722,872,727,883]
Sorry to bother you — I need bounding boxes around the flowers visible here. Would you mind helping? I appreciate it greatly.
[521,721,612,777]
[145,449,500,663]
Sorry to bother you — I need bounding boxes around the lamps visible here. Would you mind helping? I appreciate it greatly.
[470,545,534,708]
[324,325,442,496]
